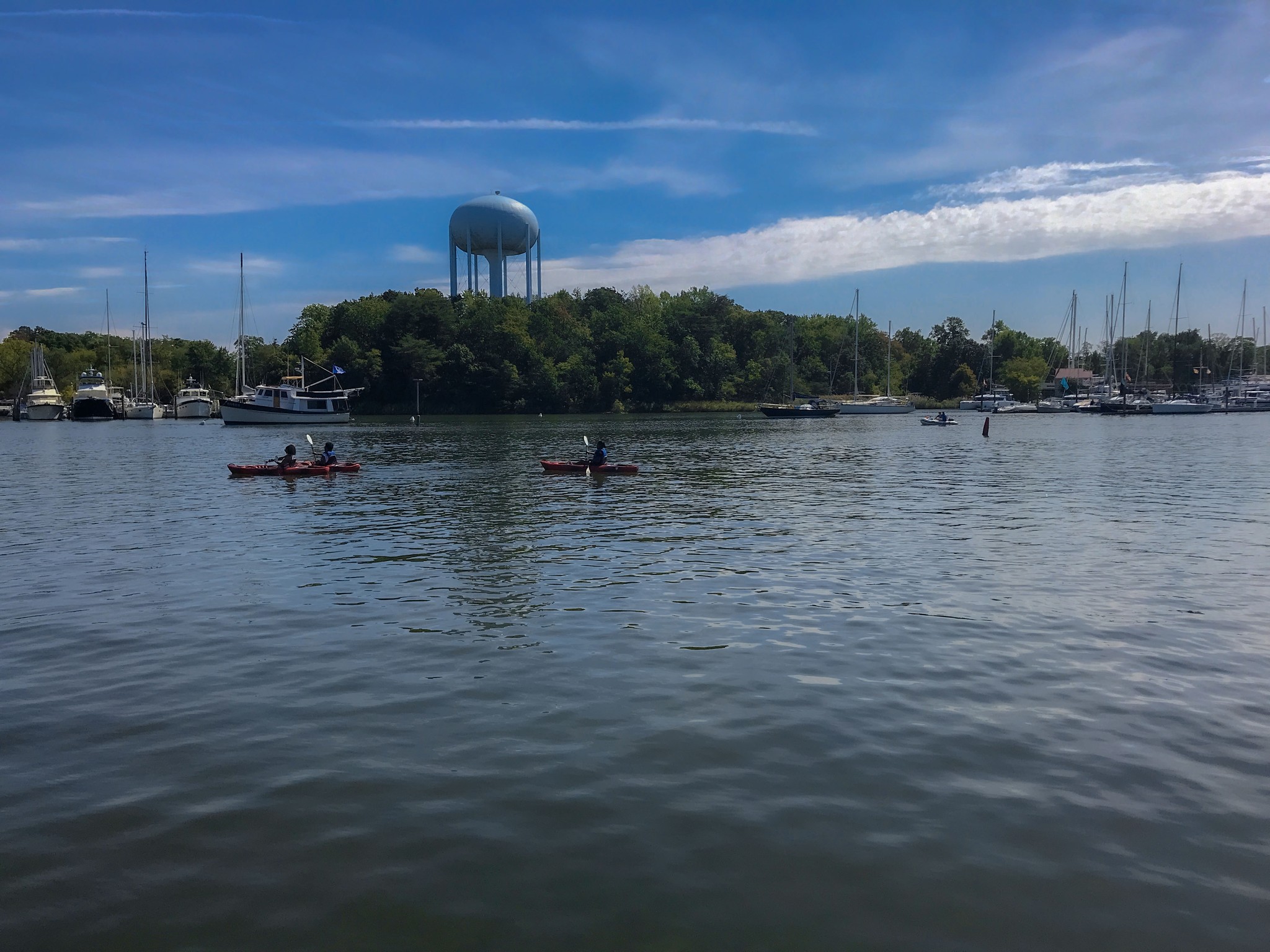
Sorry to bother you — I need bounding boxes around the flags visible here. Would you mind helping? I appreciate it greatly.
[333,365,346,374]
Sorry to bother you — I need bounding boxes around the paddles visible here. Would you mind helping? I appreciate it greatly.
[584,435,590,474]
[306,434,316,460]
[263,456,278,465]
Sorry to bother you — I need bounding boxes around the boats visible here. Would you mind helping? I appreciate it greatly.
[24,376,64,421]
[540,460,639,472]
[296,460,360,472]
[920,417,959,426]
[172,367,215,419]
[69,362,115,421]
[227,462,329,475]
[997,404,1038,413]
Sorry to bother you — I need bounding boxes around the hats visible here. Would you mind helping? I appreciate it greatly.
[598,441,606,448]
[323,442,333,449]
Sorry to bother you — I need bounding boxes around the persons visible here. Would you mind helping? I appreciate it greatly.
[568,440,607,465]
[268,444,296,468]
[934,411,948,422]
[312,442,336,466]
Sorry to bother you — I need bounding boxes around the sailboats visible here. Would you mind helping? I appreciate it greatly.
[94,289,130,416]
[755,316,841,418]
[1035,262,1270,414]
[828,287,917,414]
[960,310,1021,410]
[123,245,166,420]
[217,252,364,425]
